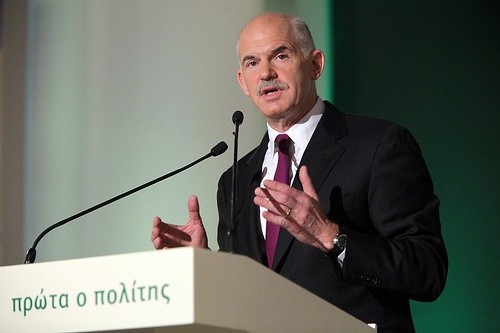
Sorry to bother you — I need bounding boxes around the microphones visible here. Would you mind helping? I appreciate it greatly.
[23,141,228,264]
[229,111,244,252]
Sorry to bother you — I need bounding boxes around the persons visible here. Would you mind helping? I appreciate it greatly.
[150,16,448,333]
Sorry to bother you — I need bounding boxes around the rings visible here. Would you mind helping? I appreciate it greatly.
[284,209,291,217]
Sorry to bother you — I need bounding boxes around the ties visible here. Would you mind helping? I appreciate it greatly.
[263,133,292,270]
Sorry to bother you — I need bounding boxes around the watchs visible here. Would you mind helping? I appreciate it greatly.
[324,229,348,258]
